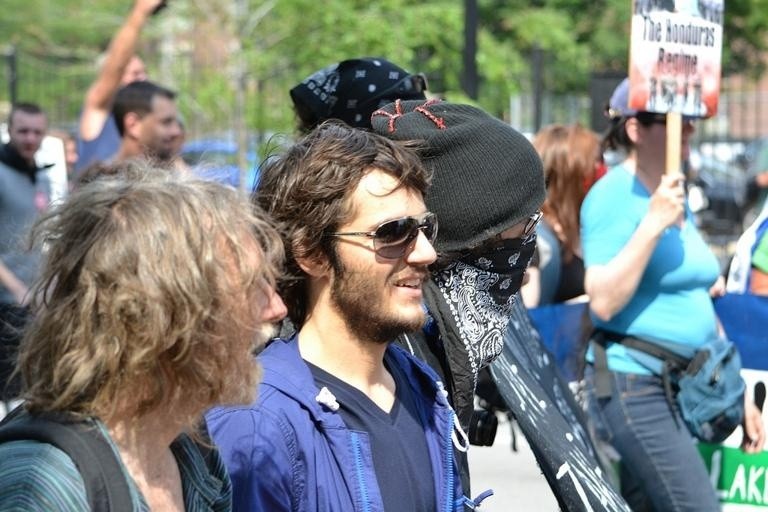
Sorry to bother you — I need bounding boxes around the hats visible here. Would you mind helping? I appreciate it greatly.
[605,74,704,120]
[372,100,547,251]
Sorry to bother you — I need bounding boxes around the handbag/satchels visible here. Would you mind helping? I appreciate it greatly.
[677,338,748,443]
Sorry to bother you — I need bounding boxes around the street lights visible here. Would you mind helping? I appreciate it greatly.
[0,32,25,107]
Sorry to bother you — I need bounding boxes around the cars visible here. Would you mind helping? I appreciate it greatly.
[682,127,768,236]
[175,137,270,189]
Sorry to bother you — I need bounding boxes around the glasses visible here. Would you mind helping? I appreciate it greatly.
[356,71,427,112]
[315,210,440,261]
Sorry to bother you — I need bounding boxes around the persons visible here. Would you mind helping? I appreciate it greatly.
[364,95,551,512]
[575,73,768,511]
[72,1,167,181]
[503,122,608,315]
[1,143,293,512]
[741,138,768,237]
[193,117,497,512]
[706,199,768,302]
[171,114,189,172]
[105,77,180,172]
[1,102,63,407]
